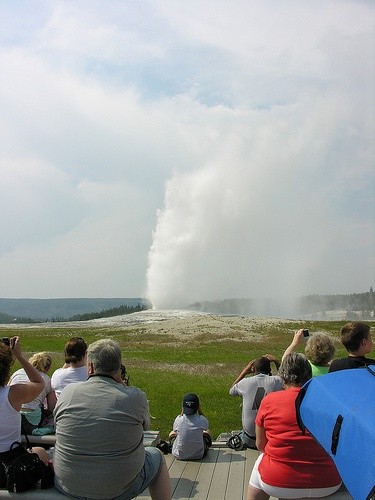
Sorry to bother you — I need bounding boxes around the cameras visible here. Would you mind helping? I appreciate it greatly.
[303,329,310,338]
[0,338,16,349]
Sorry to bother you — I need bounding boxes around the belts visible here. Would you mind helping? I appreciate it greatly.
[244,432,256,440]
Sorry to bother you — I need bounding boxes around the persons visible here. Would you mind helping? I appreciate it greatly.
[169,394,212,461]
[281,329,336,378]
[53,339,172,500]
[228,354,283,450]
[51,337,89,401]
[6,350,57,434]
[247,352,342,500]
[328,322,375,373]
[0,336,49,491]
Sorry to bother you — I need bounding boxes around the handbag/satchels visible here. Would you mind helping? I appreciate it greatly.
[4,441,55,493]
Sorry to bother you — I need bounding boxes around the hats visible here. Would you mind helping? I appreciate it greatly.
[183,394,199,414]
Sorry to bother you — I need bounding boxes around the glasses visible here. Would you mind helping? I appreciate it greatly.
[0,337,16,350]
